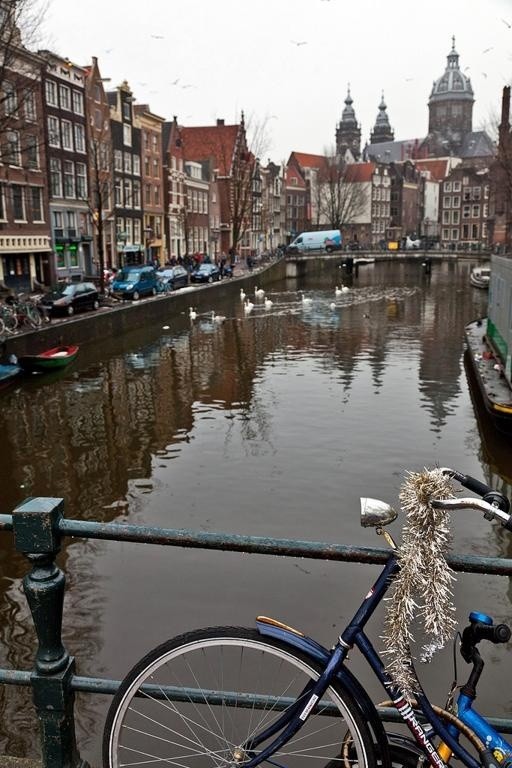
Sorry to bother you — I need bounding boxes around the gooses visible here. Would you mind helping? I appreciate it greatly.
[263,297,272,305]
[210,310,225,321]
[253,285,265,295]
[334,286,341,294]
[239,288,245,297]
[340,284,349,292]
[243,301,251,310]
[246,298,253,306]
[188,306,196,316]
[301,294,312,302]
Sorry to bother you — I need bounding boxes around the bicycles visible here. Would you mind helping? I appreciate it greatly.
[0,291,50,339]
[96,461,510,766]
[338,606,512,768]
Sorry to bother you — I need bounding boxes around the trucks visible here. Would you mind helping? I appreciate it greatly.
[285,229,343,253]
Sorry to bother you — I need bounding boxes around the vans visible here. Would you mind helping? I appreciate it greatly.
[109,265,156,303]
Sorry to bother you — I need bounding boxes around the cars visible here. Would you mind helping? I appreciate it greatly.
[121,339,167,375]
[70,363,109,394]
[155,265,189,292]
[33,280,101,319]
[190,263,223,284]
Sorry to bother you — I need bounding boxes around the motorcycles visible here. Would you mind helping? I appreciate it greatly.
[222,263,237,278]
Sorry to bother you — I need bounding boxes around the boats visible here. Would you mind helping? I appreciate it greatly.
[25,360,80,389]
[23,343,82,372]
[468,265,490,289]
[0,361,24,386]
